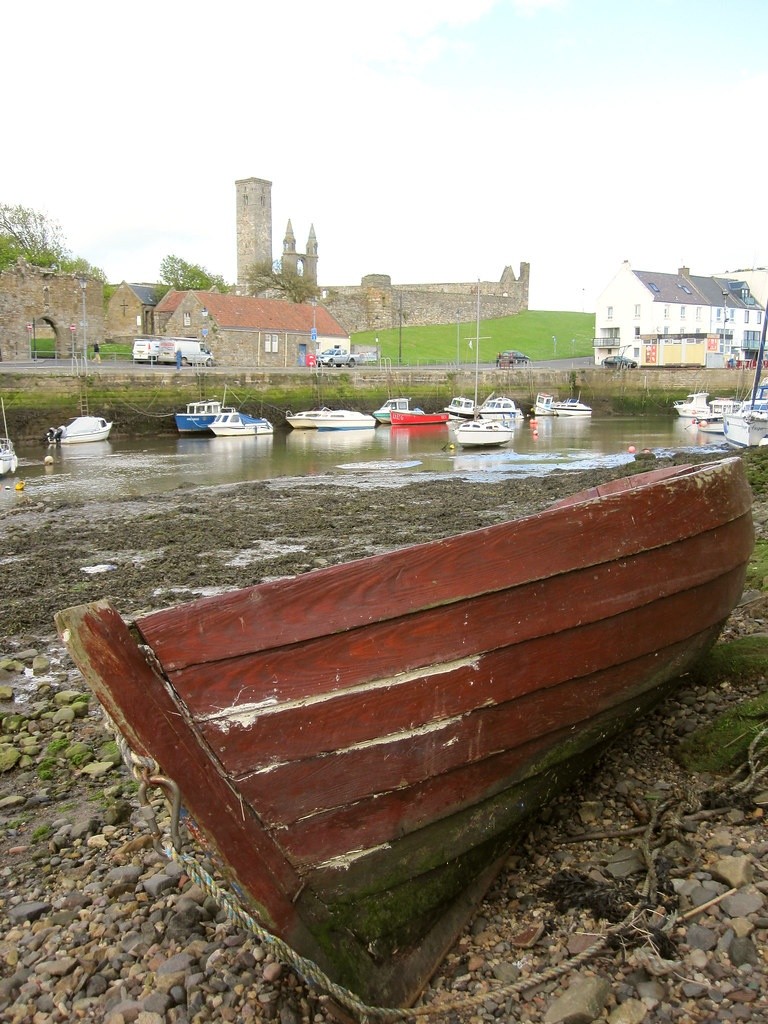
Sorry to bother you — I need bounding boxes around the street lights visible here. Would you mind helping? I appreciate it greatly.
[722,288,729,354]
[311,296,318,354]
[455,310,461,365]
[202,307,209,340]
[79,277,88,366]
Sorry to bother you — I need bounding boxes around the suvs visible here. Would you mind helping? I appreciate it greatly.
[496,350,529,367]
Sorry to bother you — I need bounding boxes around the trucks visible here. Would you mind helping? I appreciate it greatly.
[132,337,214,367]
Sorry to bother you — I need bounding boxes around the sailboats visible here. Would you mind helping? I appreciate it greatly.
[720,300,768,448]
[454,278,513,448]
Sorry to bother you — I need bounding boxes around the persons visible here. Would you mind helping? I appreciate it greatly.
[496,351,502,368]
[91,341,101,364]
[177,348,182,369]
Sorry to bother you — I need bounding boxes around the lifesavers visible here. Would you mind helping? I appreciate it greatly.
[720,398,729,400]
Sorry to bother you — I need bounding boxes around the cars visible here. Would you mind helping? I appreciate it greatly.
[604,356,637,369]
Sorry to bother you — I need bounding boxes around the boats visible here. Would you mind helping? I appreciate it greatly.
[533,392,555,415]
[285,389,450,431]
[673,388,743,433]
[551,389,592,416]
[0,397,18,475]
[208,406,274,437]
[52,455,755,1024]
[175,396,235,433]
[46,416,113,444]
[475,396,482,422]
[478,396,525,420]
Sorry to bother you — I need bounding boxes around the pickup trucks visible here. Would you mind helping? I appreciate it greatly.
[316,347,360,367]
